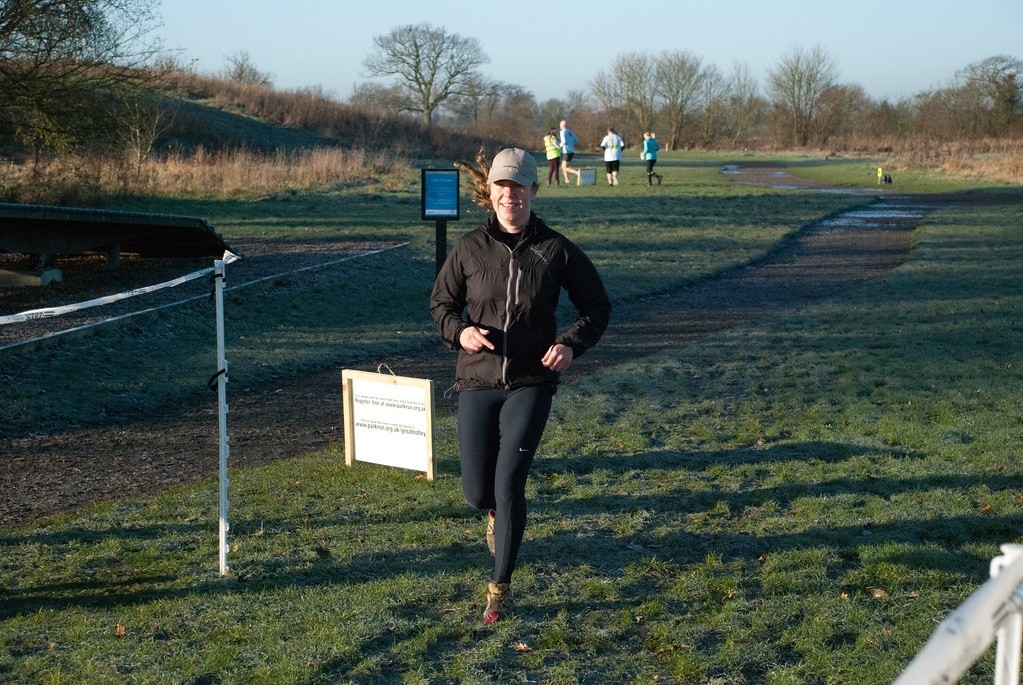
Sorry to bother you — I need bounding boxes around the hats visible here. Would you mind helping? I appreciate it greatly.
[485,148,538,187]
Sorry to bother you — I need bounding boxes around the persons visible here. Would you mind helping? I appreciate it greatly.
[543,128,562,186]
[559,121,578,183]
[431,148,612,622]
[644,132,662,186]
[601,127,625,186]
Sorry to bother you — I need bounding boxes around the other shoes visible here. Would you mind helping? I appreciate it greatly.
[609,184,613,187]
[486,511,499,556]
[647,183,652,186]
[483,583,510,621]
[657,176,662,186]
